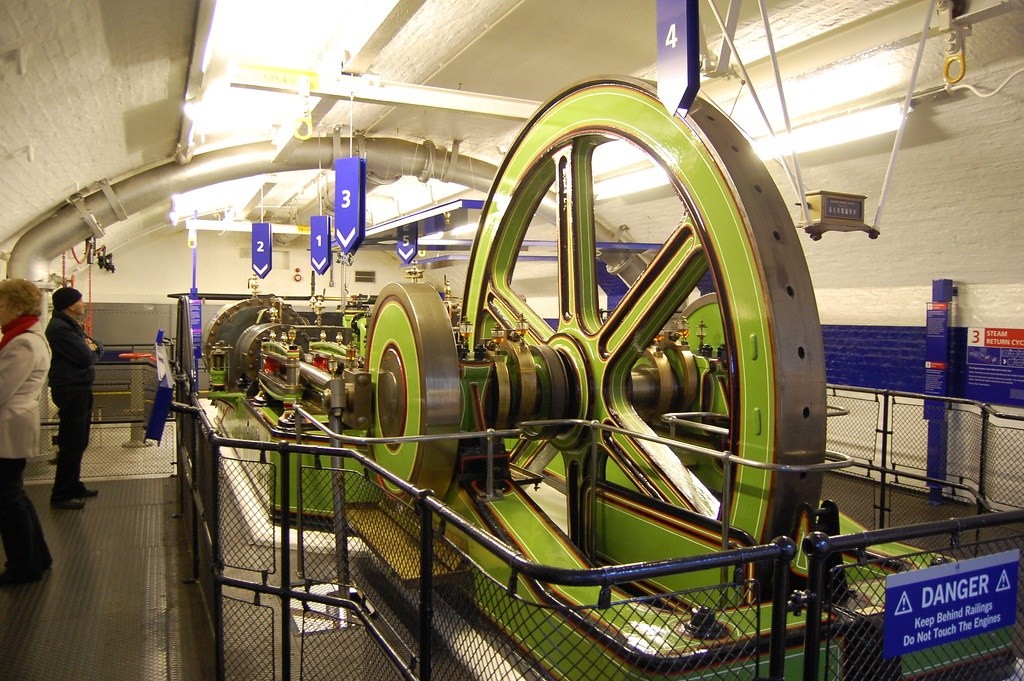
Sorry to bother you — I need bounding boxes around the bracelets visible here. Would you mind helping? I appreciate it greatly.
[93,343,98,351]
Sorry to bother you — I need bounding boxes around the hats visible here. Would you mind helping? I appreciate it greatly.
[53,287,82,310]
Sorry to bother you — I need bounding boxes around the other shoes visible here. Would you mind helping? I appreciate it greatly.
[79,485,99,496]
[50,497,85,510]
[0,555,54,585]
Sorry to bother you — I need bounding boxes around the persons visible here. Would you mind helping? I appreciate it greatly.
[0,277,53,586]
[41,286,106,510]
